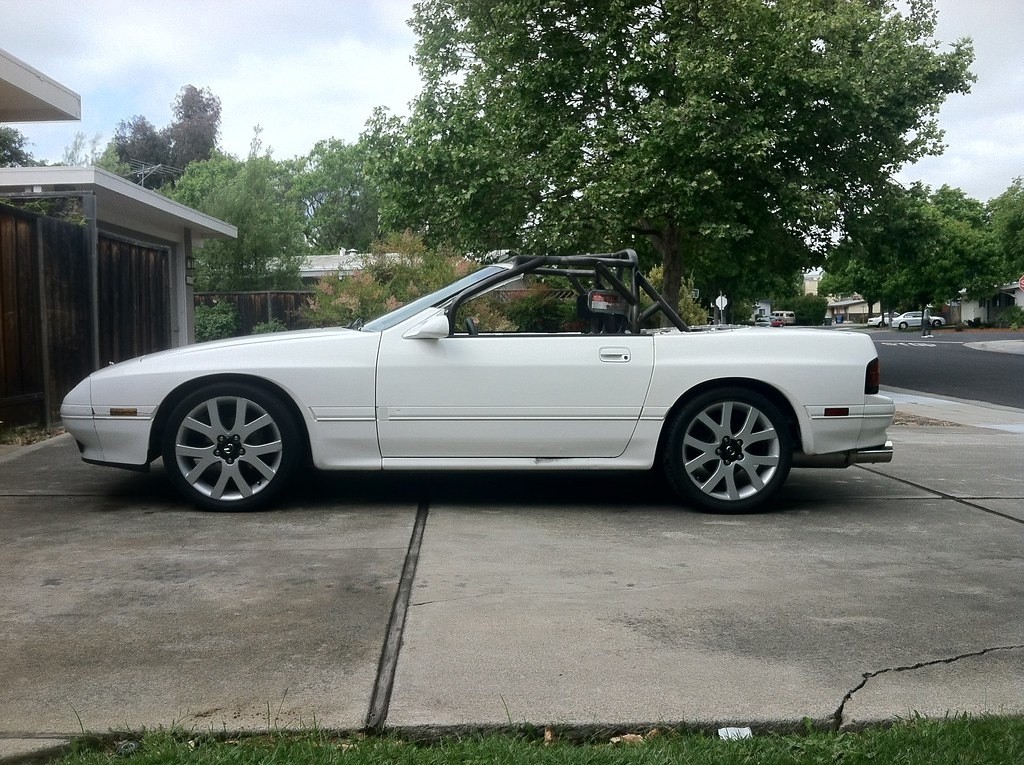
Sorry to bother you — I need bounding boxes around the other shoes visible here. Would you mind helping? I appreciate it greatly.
[921,336,928,339]
[927,335,933,337]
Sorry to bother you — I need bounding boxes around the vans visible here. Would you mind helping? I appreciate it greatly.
[769,310,796,325]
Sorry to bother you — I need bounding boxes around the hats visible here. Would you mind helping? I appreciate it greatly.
[926,304,934,308]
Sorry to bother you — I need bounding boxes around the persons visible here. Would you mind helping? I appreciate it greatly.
[921,307,933,338]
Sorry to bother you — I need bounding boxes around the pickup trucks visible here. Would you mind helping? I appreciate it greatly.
[55,248,895,515]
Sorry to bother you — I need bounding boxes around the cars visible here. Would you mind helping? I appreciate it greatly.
[892,311,946,331]
[756,315,770,322]
[770,318,784,327]
[867,312,900,328]
